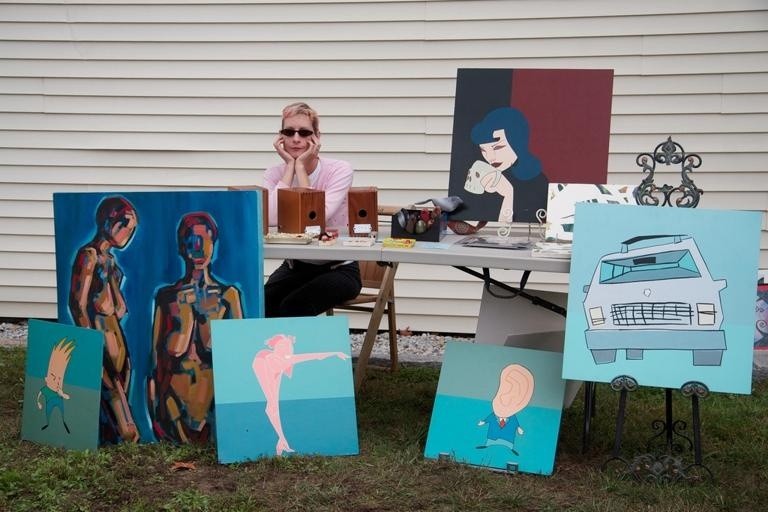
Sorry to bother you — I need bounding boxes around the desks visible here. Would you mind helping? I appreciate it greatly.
[262,227,595,453]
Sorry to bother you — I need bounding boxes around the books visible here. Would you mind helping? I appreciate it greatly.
[530,241,572,262]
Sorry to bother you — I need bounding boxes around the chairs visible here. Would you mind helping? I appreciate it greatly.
[327,205,415,397]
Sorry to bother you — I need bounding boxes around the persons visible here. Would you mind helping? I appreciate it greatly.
[259,102,364,320]
[144,213,244,444]
[67,196,141,447]
[455,108,549,225]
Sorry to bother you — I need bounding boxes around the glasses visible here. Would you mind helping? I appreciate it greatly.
[280,128,313,137]
